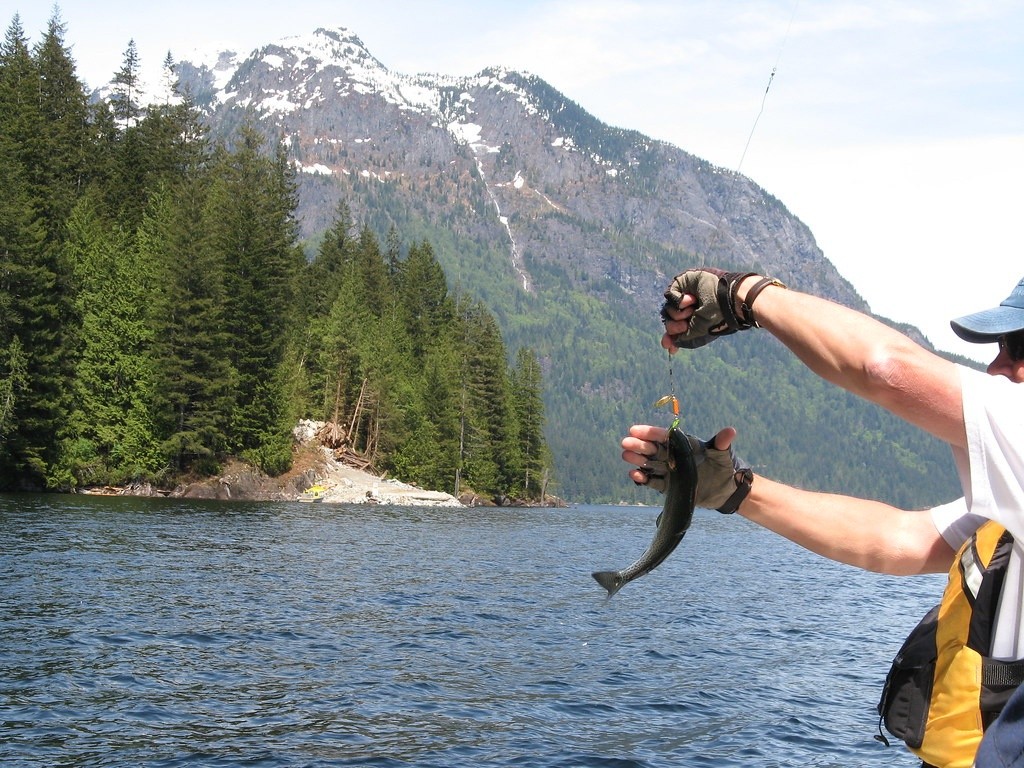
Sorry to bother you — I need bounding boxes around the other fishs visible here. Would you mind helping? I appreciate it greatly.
[592,426,699,607]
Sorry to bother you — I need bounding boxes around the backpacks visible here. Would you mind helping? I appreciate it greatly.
[874,518,1024,768]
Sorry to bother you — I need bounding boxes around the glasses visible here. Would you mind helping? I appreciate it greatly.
[993,328,1024,362]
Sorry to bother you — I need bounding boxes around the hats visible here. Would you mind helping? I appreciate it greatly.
[950,277,1024,344]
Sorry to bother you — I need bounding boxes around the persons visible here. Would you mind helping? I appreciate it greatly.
[620,269,1024,768]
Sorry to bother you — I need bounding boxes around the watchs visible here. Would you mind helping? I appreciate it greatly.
[740,275,789,328]
